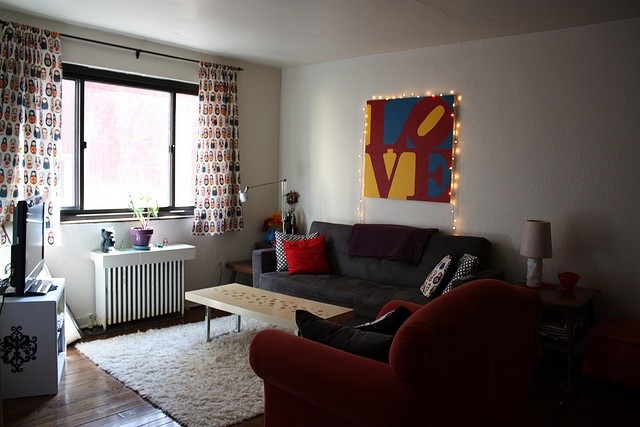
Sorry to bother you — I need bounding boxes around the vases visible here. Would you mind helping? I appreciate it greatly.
[265,227,275,243]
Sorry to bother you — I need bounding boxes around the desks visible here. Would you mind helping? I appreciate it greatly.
[513,283,602,390]
[226,260,253,285]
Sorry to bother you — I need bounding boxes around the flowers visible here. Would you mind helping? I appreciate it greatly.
[262,211,282,233]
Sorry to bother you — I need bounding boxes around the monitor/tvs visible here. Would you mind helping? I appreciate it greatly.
[7,193,51,294]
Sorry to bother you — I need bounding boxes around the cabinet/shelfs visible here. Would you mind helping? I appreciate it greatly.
[2,278,66,398]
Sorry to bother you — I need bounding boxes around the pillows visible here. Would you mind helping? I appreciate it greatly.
[282,231,331,275]
[294,309,394,364]
[420,255,452,298]
[275,231,329,274]
[355,306,411,333]
[443,254,480,291]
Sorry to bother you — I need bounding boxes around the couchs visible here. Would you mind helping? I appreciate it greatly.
[249,279,545,427]
[252,221,505,322]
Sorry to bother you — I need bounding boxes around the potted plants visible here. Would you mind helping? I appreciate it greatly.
[128,191,158,252]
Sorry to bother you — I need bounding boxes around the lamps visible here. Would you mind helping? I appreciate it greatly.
[239,179,288,235]
[519,220,552,285]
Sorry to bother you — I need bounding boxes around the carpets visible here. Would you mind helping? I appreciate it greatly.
[75,314,299,427]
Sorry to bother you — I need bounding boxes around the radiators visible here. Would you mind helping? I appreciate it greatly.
[88,259,185,329]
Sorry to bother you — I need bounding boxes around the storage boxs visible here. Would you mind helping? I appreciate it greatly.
[584,314,639,389]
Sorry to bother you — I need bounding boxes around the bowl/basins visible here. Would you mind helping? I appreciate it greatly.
[557,271,581,290]
